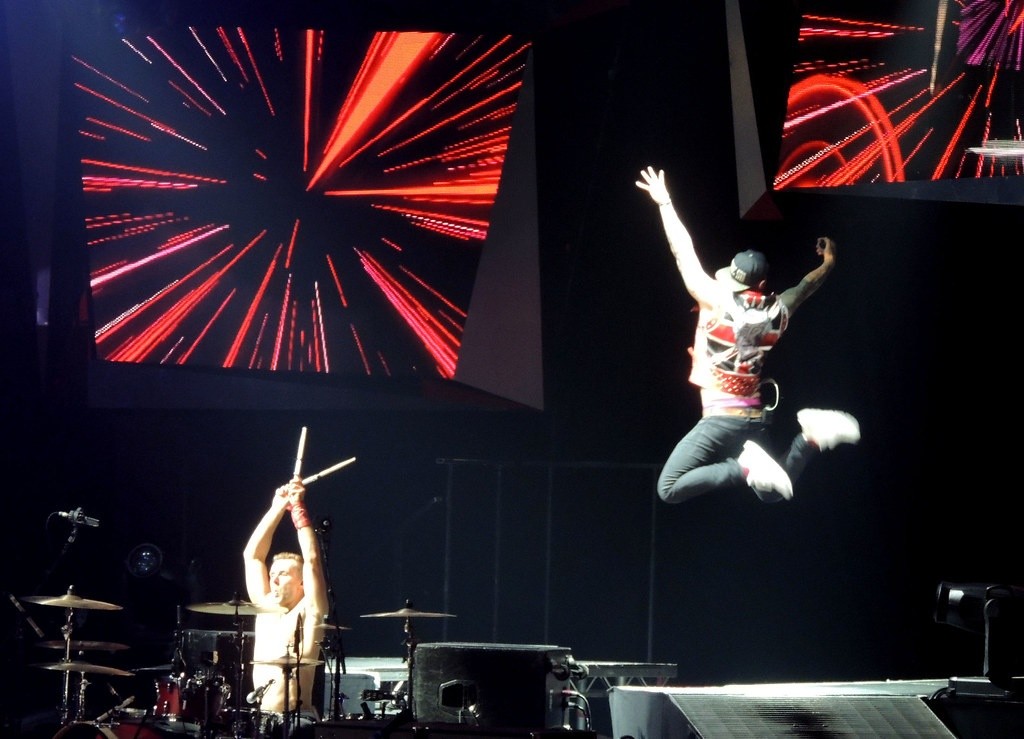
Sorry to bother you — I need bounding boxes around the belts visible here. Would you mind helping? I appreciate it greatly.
[702,407,762,417]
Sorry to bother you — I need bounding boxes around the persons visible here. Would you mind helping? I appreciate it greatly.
[635,166,860,504]
[243,476,328,733]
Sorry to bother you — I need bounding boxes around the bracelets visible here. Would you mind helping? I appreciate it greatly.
[658,202,671,206]
[287,502,311,529]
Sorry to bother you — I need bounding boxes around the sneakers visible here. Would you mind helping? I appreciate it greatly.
[738,440,793,501]
[797,408,861,452]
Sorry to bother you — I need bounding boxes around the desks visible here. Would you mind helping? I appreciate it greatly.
[573,660,679,729]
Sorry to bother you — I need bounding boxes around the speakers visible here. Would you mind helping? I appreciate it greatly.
[411,643,573,732]
[180,628,256,709]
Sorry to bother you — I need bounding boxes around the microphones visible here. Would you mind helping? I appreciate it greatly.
[8,594,45,639]
[106,682,123,706]
[293,614,301,653]
[59,511,100,527]
[320,519,332,530]
[246,679,275,704]
[95,697,136,723]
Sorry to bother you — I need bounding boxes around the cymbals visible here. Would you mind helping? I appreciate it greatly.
[300,623,353,631]
[41,663,135,676]
[360,608,457,618]
[249,653,326,669]
[184,599,289,616]
[20,593,124,610]
[34,640,130,652]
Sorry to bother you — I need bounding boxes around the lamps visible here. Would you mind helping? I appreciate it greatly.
[127,543,162,576]
[936,583,1024,696]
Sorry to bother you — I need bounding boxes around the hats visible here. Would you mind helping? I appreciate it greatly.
[715,249,769,292]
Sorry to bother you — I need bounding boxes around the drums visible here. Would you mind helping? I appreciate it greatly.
[52,720,164,739]
[151,668,231,735]
[111,705,165,724]
[208,708,278,739]
